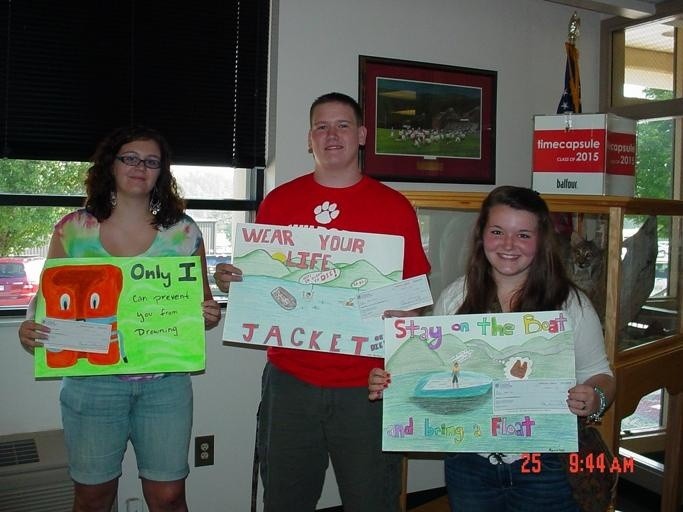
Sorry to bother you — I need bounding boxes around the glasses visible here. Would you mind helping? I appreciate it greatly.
[116,156,161,169]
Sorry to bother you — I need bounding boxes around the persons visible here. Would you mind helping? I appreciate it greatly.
[368,185,617,512]
[213,93,433,512]
[18,120,221,512]
[397,124,473,147]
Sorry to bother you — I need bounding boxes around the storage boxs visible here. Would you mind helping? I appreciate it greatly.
[528,109,640,199]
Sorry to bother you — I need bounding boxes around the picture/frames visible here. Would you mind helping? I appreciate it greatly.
[357,54,499,188]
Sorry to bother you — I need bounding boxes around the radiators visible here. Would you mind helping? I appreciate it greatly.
[0,430,78,509]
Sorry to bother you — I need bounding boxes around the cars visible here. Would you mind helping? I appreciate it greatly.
[204,253,231,297]
[0,254,46,316]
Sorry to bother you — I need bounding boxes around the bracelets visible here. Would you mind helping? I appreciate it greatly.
[590,383,609,422]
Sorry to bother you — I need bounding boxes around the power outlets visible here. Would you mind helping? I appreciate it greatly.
[194,433,214,466]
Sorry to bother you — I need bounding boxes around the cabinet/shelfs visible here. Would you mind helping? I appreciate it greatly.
[396,193,682,511]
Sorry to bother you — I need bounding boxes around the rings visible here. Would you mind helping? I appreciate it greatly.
[582,402,586,410]
